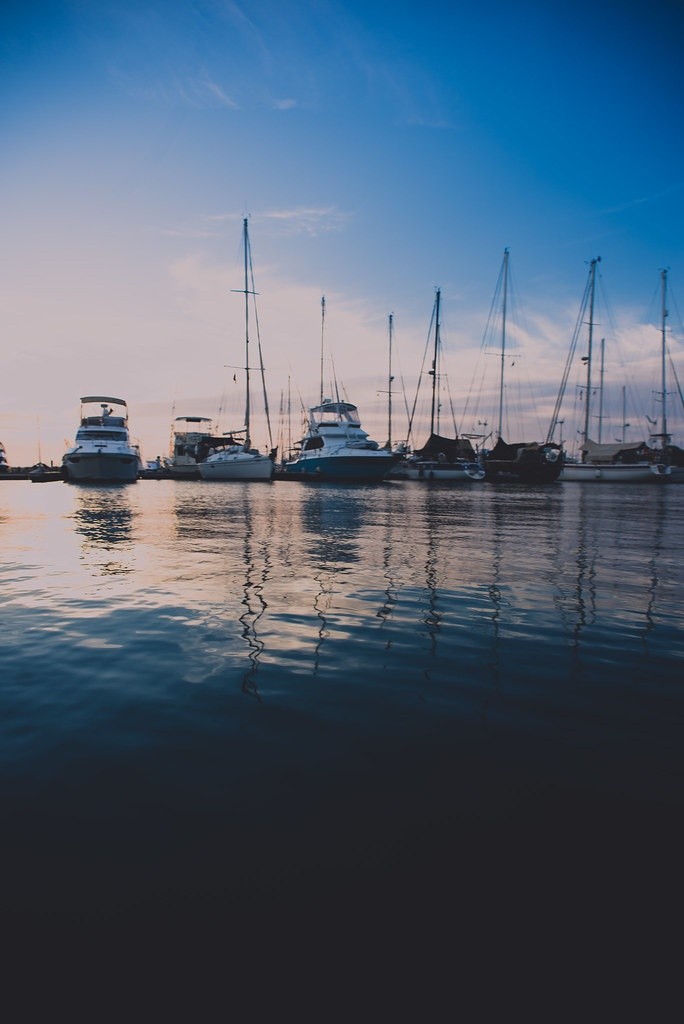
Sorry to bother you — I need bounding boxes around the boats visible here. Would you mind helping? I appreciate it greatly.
[27,463,65,484]
[60,394,141,482]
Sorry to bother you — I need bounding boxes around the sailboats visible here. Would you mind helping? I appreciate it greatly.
[142,215,684,479]
